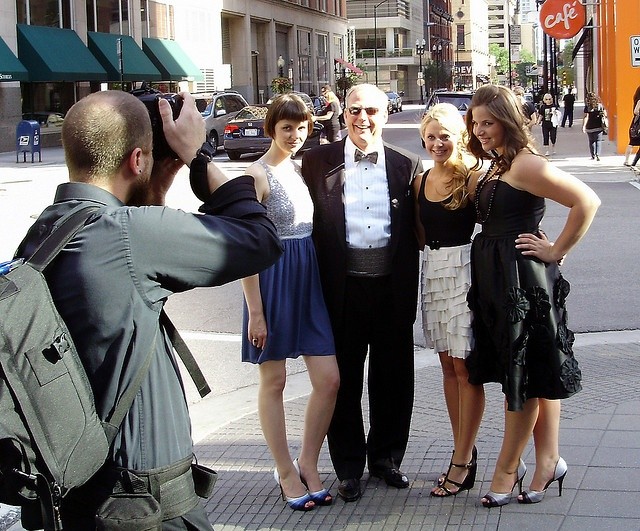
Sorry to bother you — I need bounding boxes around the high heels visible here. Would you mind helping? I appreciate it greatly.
[517,457,568,504]
[273,466,318,511]
[438,444,478,485]
[482,456,528,508]
[292,457,333,506]
[430,457,478,497]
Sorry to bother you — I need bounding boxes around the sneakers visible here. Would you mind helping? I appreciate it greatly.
[596,153,599,159]
[591,156,594,159]
[546,151,549,155]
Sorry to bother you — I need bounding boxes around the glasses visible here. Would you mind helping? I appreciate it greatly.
[348,106,380,115]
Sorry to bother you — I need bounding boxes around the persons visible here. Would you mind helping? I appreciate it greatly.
[301,84,422,499]
[537,94,561,156]
[437,84,601,507]
[584,95,604,161]
[561,86,576,126]
[624,84,640,167]
[627,146,640,169]
[312,92,339,141]
[512,86,537,135]
[244,94,333,509]
[1,92,282,531]
[411,104,486,496]
[320,85,330,95]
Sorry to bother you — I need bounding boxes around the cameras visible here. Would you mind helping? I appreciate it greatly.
[129,85,184,159]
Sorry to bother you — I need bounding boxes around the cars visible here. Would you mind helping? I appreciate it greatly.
[223,104,324,160]
[385,91,403,113]
[311,96,344,124]
[525,93,533,100]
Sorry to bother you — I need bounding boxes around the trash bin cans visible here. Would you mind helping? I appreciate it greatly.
[16,120,41,163]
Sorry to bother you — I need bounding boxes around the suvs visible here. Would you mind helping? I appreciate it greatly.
[422,88,477,149]
[190,90,249,156]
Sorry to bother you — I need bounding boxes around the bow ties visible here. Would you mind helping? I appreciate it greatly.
[354,148,379,164]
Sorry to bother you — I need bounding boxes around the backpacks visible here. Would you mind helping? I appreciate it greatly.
[0,203,212,506]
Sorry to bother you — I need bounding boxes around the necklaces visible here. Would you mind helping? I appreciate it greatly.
[475,159,504,224]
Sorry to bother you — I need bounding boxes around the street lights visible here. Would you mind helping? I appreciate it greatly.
[277,54,284,76]
[432,44,442,88]
[342,64,346,106]
[415,38,427,103]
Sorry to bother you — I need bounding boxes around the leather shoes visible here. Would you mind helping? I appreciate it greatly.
[369,466,410,489]
[337,479,361,502]
